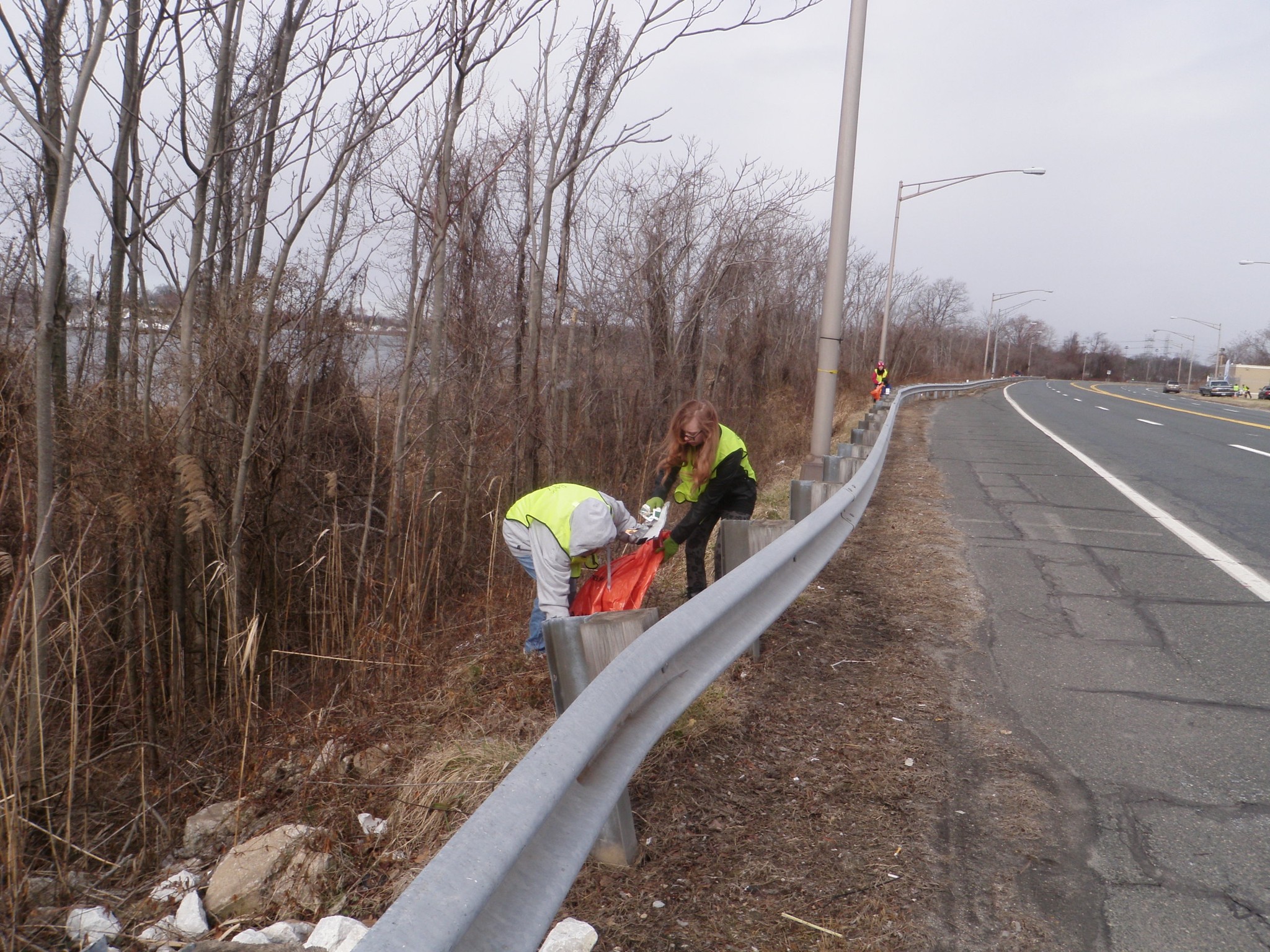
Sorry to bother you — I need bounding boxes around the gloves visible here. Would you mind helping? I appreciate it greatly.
[655,537,680,563]
[645,497,665,514]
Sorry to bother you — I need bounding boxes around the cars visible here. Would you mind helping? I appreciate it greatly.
[1257,386,1270,400]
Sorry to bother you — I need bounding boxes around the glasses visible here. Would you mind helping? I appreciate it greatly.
[683,431,703,441]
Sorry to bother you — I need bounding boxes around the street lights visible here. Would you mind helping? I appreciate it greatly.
[982,289,1054,380]
[1144,315,1222,393]
[878,167,1047,363]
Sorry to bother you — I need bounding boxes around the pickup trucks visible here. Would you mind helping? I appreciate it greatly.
[1198,380,1235,397]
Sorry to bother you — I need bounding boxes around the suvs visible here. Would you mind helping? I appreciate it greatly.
[1163,380,1180,394]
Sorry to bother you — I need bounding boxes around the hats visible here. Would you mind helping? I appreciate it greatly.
[877,361,885,368]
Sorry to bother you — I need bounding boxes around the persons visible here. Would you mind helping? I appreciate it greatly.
[502,482,672,653]
[1242,384,1252,399]
[644,400,758,603]
[871,361,889,403]
[1233,384,1239,397]
